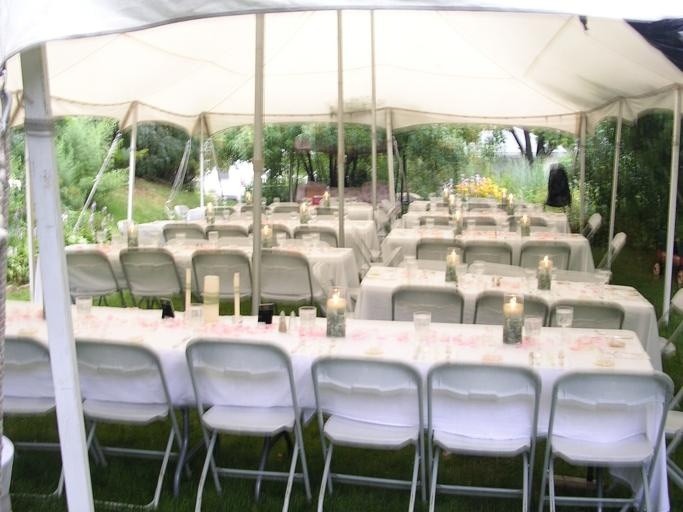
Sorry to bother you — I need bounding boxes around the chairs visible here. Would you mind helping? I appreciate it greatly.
[3,334,99,496]
[185,337,312,512]
[76,338,191,509]
[312,356,426,512]
[427,362,542,511]
[604,387,683,512]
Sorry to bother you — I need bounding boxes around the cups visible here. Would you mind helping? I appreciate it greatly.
[174,208,322,248]
[467,220,476,231]
[412,312,431,338]
[426,218,434,233]
[525,316,541,346]
[298,306,316,333]
[188,302,201,321]
[76,297,91,311]
[556,306,573,342]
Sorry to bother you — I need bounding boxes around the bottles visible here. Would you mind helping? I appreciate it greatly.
[280,310,297,331]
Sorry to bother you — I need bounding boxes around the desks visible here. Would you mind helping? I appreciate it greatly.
[3,298,671,512]
